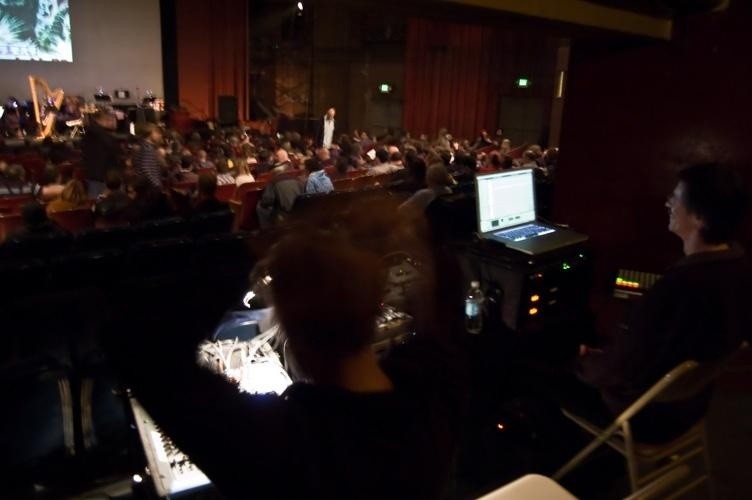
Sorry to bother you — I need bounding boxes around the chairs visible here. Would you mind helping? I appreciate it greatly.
[2,120,560,327]
[545,344,751,499]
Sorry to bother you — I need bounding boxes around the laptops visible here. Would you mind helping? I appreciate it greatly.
[474,168,588,256]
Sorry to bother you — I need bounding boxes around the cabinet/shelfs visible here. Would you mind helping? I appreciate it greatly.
[460,232,592,346]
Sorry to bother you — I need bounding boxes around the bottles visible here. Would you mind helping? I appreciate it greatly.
[464,281,484,334]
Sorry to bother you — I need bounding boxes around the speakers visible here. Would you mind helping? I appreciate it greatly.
[219,95,237,127]
